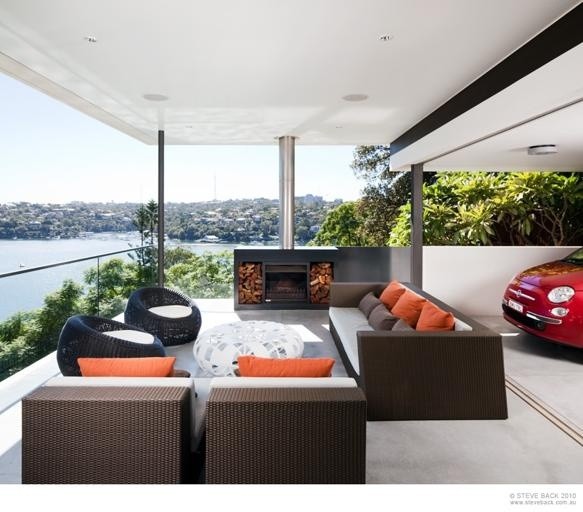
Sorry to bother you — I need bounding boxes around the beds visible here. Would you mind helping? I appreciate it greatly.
[193,321,303,376]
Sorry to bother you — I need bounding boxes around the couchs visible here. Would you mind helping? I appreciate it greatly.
[55,314,167,376]
[207,376,365,485]
[125,288,202,344]
[329,282,513,420]
[23,377,204,485]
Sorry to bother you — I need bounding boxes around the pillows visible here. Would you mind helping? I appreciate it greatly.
[391,320,417,331]
[379,281,403,304]
[417,300,454,329]
[391,289,423,323]
[236,355,335,377]
[77,355,176,377]
[359,292,378,314]
[369,306,396,331]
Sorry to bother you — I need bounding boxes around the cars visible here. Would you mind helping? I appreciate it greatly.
[500,248,582,354]
